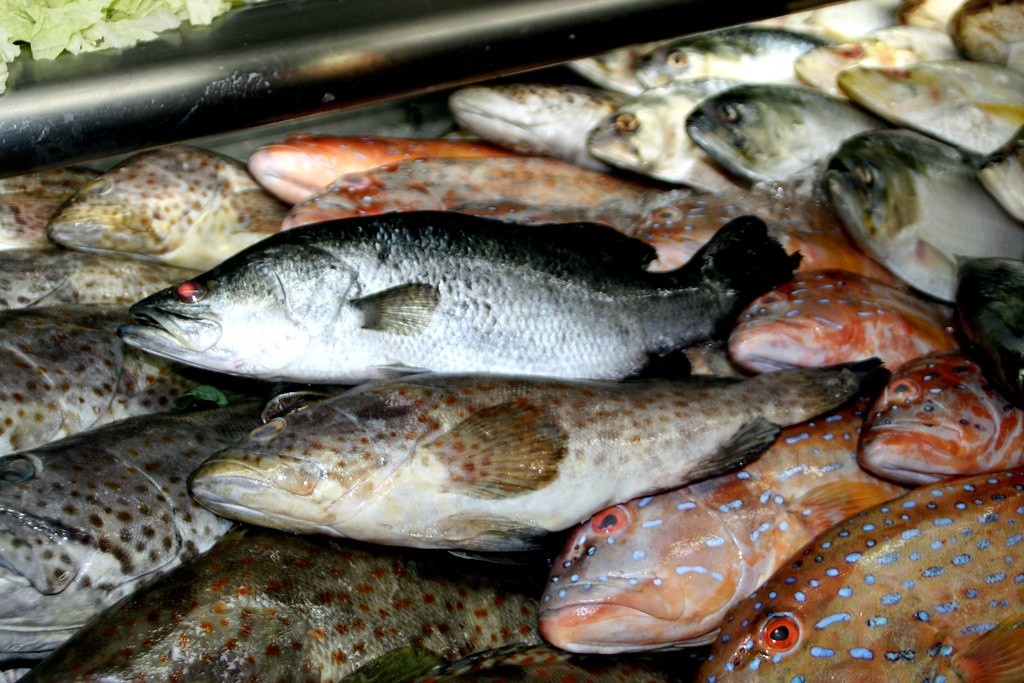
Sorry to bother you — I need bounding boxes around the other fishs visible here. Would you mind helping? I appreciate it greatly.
[0,0,1024,683]
